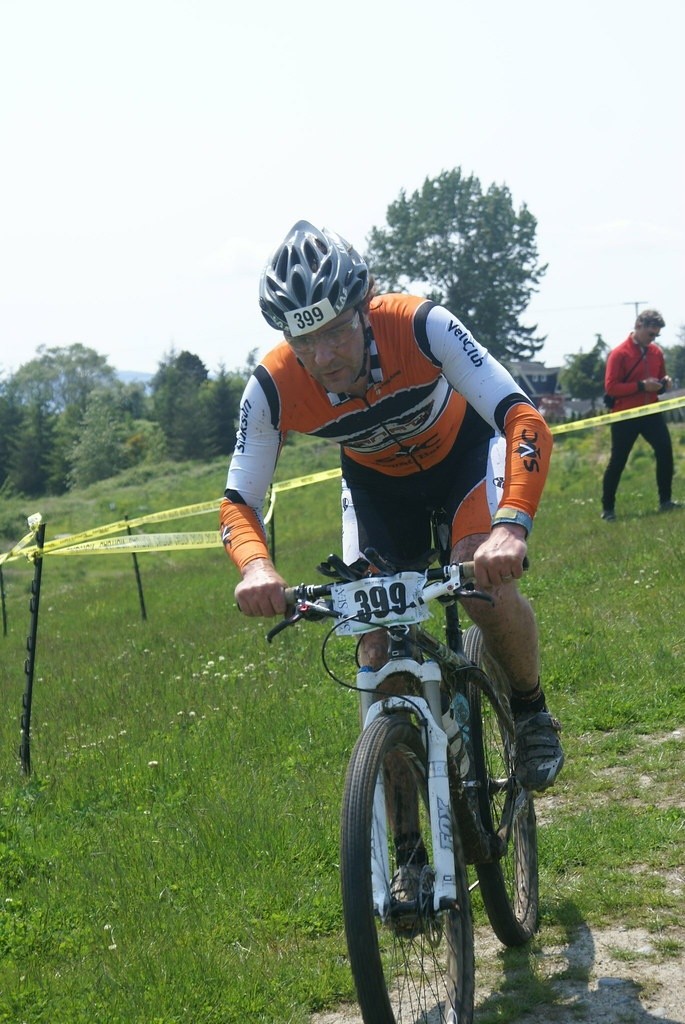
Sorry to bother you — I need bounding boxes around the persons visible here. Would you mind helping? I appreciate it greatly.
[600,310,681,520]
[217,217,565,930]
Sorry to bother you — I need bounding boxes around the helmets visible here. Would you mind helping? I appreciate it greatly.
[258,220,369,331]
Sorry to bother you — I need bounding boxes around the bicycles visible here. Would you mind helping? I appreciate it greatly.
[231,553,542,1024]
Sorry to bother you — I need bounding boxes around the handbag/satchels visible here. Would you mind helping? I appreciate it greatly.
[603,392,615,408]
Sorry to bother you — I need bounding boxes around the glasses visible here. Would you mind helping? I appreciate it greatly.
[283,309,359,353]
[643,325,661,338]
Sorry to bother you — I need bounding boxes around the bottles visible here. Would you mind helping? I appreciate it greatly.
[437,688,471,777]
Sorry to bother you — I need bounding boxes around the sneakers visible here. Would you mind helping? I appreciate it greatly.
[373,863,432,939]
[512,703,564,791]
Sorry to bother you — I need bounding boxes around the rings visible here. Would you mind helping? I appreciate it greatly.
[501,574,512,581]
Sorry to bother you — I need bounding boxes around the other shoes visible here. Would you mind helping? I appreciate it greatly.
[601,508,617,522]
[658,502,684,512]
